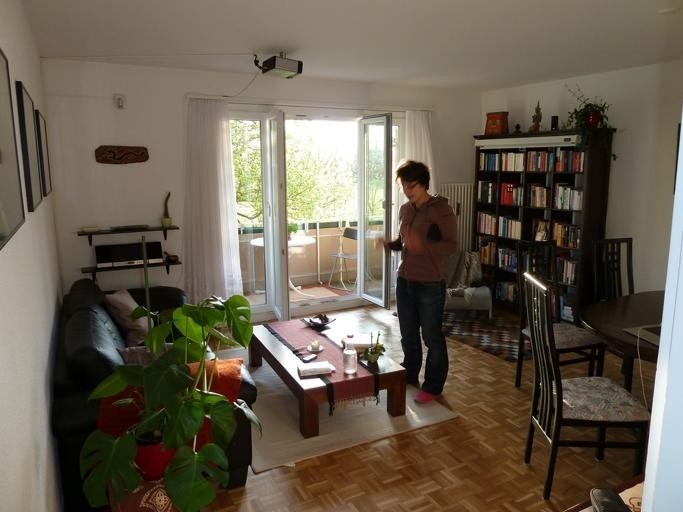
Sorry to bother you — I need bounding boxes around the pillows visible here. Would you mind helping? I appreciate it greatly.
[104,288,155,349]
[116,342,176,366]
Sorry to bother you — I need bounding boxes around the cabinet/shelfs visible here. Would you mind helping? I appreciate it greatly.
[472,127,618,326]
[78,223,184,281]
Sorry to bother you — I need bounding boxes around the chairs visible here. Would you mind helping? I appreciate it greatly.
[521,269,651,511]
[515,244,606,393]
[443,252,493,322]
[582,238,635,373]
[328,227,372,293]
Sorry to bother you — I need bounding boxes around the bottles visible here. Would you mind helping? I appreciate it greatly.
[343,334,357,375]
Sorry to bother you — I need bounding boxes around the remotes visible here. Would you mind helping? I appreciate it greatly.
[303,353,318,362]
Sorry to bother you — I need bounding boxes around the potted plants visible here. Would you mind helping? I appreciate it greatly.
[81,294,264,512]
[560,83,609,128]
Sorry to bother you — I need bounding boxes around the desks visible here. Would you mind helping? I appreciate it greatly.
[249,234,317,302]
[581,291,666,399]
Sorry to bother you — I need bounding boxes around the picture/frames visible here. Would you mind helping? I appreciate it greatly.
[15,80,44,213]
[0,49,27,253]
[35,110,53,198]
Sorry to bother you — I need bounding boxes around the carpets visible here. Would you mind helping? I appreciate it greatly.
[392,305,535,364]
[210,343,460,475]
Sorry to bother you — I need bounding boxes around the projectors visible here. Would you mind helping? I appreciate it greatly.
[262,56,304,79]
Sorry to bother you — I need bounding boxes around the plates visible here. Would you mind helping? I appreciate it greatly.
[301,317,339,327]
[307,346,324,352]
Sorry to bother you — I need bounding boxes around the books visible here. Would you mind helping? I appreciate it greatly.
[475,146,585,323]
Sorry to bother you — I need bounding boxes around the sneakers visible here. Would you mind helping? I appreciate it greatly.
[414,389,443,404]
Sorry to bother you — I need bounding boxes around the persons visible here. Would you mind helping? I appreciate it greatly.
[374,159,458,405]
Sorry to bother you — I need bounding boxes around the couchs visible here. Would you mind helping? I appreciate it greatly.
[51,279,258,489]
